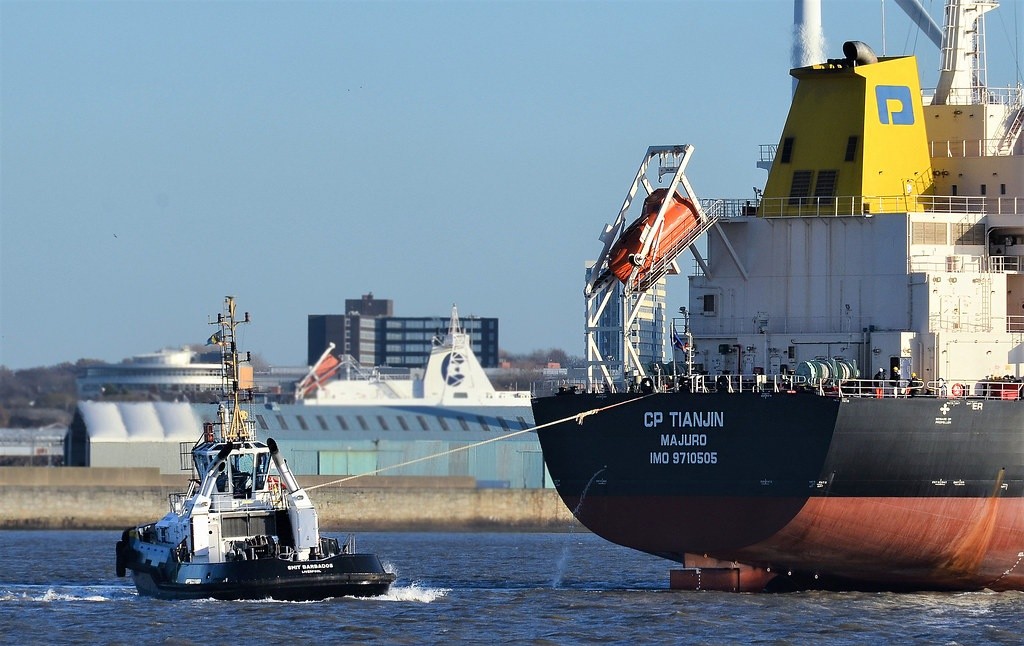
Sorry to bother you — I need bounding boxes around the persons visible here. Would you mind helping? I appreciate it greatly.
[889,367,900,387]
[874,368,886,387]
[909,373,919,397]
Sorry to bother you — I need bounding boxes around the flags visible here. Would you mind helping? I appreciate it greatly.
[205,330,222,346]
[674,334,687,353]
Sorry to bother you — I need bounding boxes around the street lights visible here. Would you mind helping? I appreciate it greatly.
[29,400,37,465]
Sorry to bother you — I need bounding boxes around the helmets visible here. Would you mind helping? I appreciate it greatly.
[911,373,917,377]
[878,368,884,372]
[893,367,899,371]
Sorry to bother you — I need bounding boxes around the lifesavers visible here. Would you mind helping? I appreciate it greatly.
[952,384,963,396]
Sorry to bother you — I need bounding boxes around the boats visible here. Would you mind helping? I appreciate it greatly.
[115,295,398,600]
[284,301,532,406]
[528,0,1024,595]
[290,353,343,396]
[606,187,701,290]
